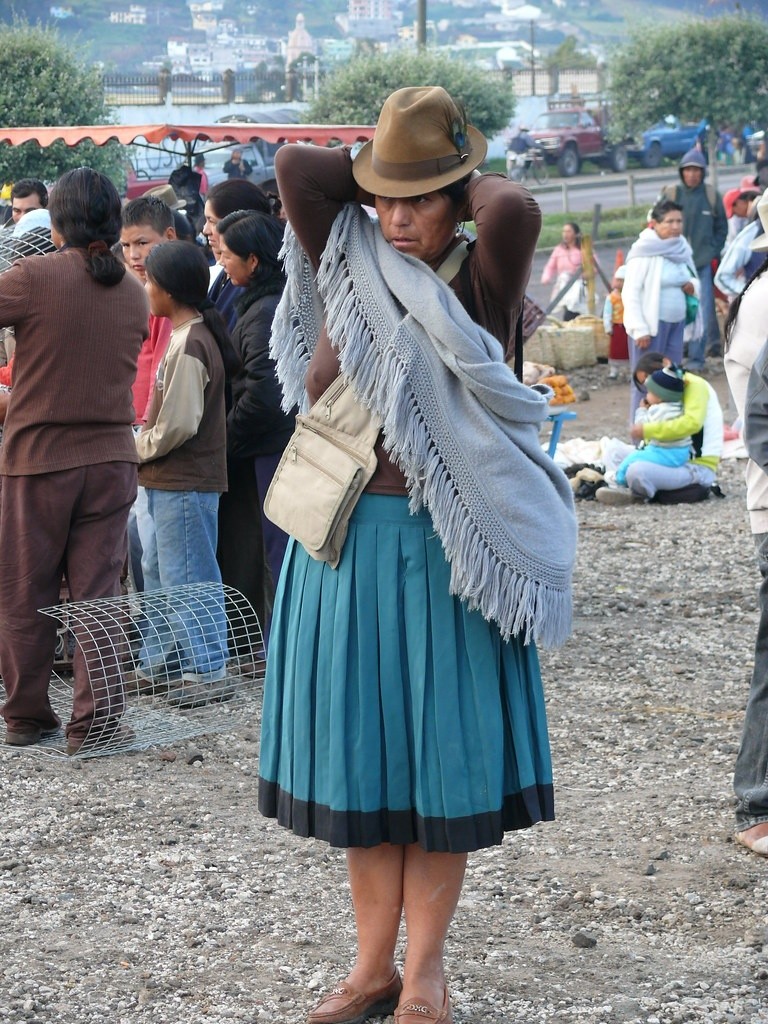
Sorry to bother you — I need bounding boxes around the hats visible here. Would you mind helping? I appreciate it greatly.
[748,186,768,252]
[351,84,488,198]
[642,362,686,402]
[141,184,187,210]
[614,265,626,279]
[724,190,743,220]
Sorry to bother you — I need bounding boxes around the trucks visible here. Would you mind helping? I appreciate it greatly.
[187,104,340,197]
[629,95,759,169]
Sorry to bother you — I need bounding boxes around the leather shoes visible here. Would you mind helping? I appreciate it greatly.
[310,967,453,1024]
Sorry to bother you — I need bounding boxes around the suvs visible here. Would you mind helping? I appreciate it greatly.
[524,95,628,176]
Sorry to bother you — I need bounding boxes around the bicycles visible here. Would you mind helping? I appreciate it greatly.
[509,144,550,185]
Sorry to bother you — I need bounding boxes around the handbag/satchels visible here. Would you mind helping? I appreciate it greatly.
[261,372,382,569]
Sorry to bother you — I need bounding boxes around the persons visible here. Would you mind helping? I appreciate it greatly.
[273,86,542,1023]
[1,147,303,706]
[1,165,149,757]
[541,222,597,322]
[595,111,767,857]
[505,123,544,180]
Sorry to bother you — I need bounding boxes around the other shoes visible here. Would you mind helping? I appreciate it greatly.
[6,716,63,746]
[237,657,266,678]
[67,724,136,756]
[596,486,646,509]
[165,680,236,708]
[124,670,165,696]
[736,824,768,857]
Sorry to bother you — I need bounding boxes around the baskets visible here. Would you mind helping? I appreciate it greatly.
[523,314,610,370]
